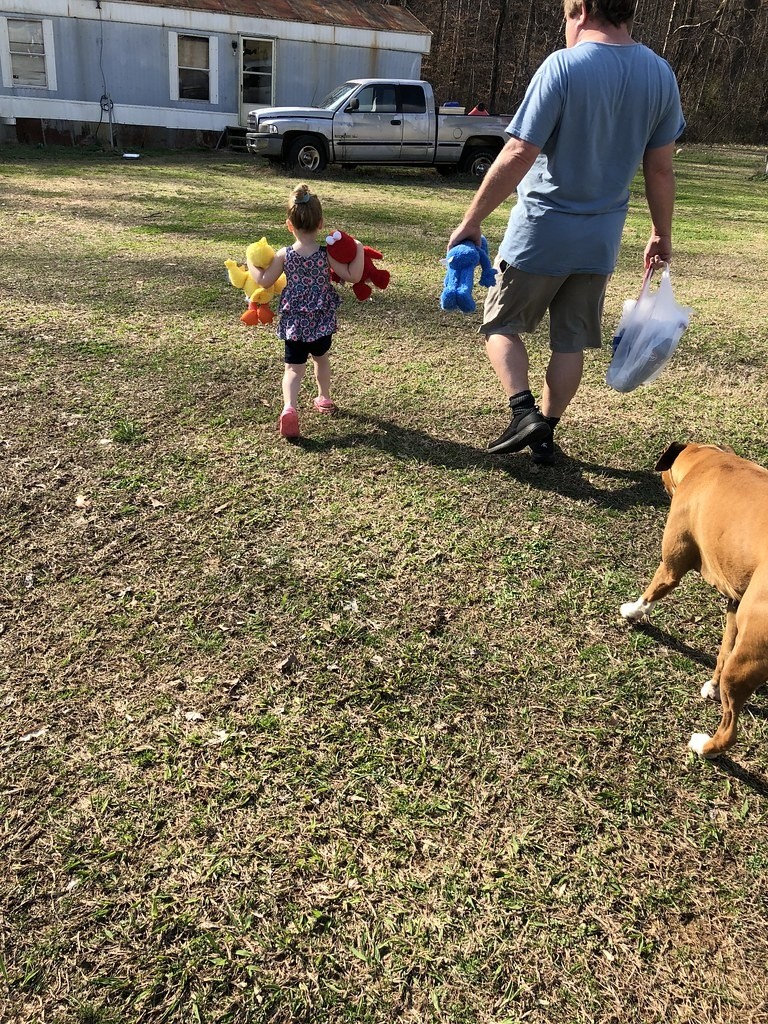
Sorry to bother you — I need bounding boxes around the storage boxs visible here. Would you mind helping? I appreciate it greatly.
[439,106,465,114]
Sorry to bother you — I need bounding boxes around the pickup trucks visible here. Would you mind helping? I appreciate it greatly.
[246,79,515,181]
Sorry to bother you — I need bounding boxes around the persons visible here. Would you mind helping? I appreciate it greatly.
[246,183,366,440]
[444,0,687,458]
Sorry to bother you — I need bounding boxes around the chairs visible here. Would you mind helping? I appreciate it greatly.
[371,97,383,112]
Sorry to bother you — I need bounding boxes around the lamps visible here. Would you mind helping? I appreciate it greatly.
[232,41,237,56]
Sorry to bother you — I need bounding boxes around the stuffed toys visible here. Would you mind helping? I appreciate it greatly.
[223,237,287,326]
[439,234,499,315]
[325,229,391,301]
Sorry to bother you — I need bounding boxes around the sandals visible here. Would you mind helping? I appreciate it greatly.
[314,397,334,412]
[280,407,300,437]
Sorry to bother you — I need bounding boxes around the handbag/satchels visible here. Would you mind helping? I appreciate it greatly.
[606,257,692,392]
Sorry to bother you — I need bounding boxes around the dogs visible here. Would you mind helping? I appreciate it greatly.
[624,441,768,758]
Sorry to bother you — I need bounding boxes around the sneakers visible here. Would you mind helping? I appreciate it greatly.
[488,407,548,454]
[529,434,556,465]
